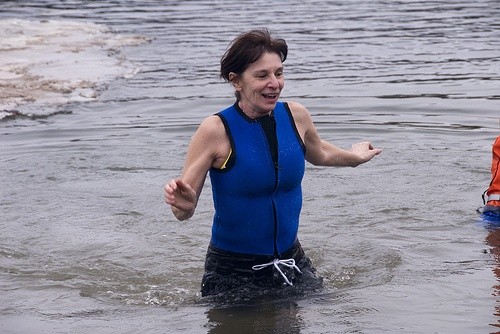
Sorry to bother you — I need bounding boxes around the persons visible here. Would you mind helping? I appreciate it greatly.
[164,27,383,304]
[483,135,500,211]
